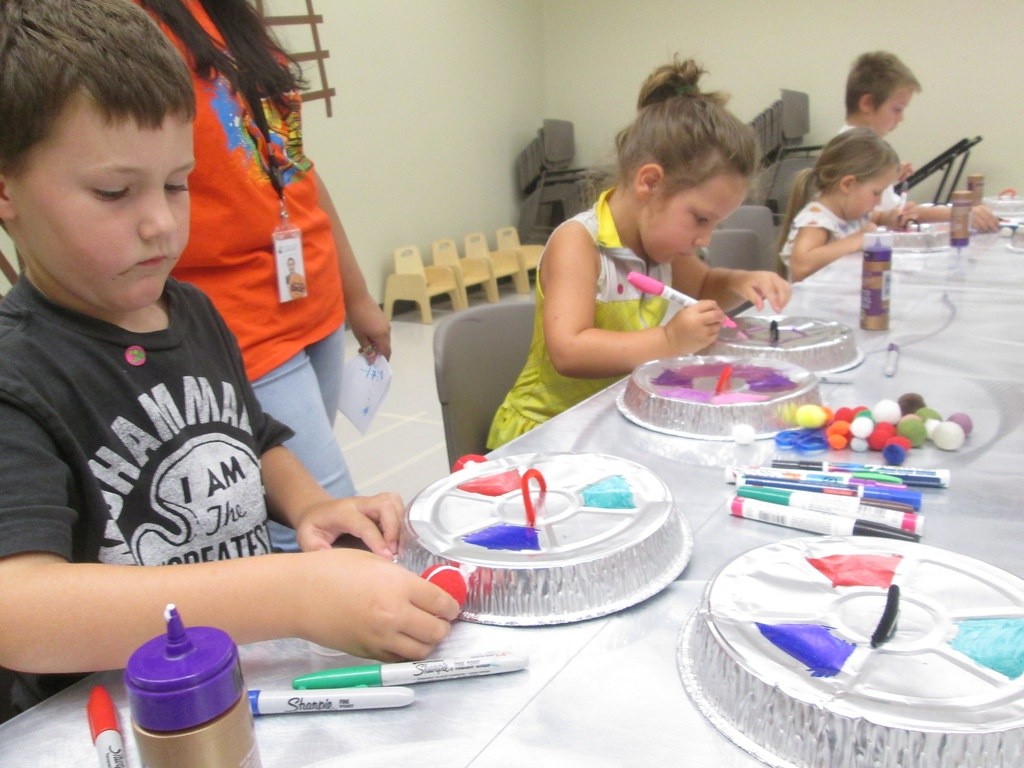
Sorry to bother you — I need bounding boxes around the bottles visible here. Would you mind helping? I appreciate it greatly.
[121,602,260,768]
[859,236,893,331]
[949,190,973,246]
[968,174,983,201]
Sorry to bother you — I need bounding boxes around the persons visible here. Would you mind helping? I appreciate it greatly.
[839,49,1002,233]
[484,49,794,452]
[778,127,901,284]
[0,0,462,726]
[285,258,305,300]
[130,0,392,554]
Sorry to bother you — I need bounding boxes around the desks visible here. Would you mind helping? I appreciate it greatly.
[0,202,1024,768]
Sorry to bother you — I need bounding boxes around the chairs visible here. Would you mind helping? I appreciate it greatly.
[494,225,545,295]
[463,231,528,302]
[382,243,463,326]
[749,85,826,227]
[431,237,498,311]
[514,118,606,245]
[433,301,536,474]
[706,227,764,318]
[714,204,781,274]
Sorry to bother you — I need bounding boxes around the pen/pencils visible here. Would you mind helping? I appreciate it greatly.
[730,461,946,541]
[243,688,416,715]
[898,180,908,215]
[86,686,129,768]
[292,655,521,687]
[627,271,737,330]
